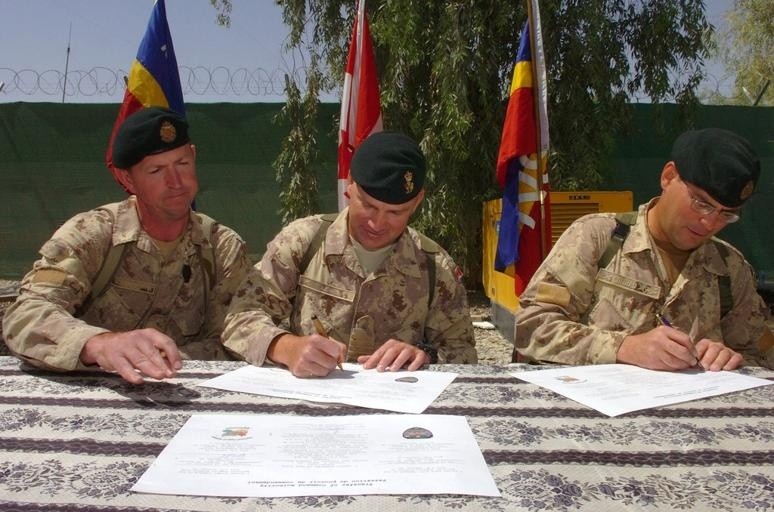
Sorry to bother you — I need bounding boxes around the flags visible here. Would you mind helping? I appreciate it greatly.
[489,14,554,302]
[335,1,386,218]
[103,0,195,208]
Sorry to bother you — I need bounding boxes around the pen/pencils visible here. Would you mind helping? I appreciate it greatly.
[656,313,705,371]
[311,314,345,373]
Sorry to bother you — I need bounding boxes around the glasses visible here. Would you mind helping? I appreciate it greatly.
[677,183,741,224]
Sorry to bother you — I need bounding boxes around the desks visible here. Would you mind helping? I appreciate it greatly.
[0,363,774,512]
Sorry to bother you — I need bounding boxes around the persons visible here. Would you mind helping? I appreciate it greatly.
[506,126,774,376]
[0,105,256,388]
[216,127,480,378]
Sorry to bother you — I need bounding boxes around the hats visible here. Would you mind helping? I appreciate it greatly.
[671,126,762,207]
[111,106,190,169]
[350,131,426,205]
